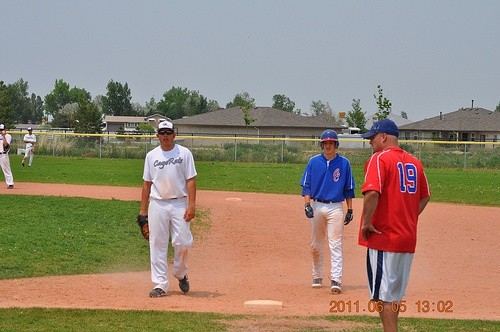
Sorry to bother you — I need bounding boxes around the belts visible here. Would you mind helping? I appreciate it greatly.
[315,198,337,203]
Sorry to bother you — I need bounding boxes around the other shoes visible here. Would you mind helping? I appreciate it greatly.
[22,159,25,163]
[29,164,32,167]
[331,280,341,292]
[8,185,13,189]
[312,279,322,287]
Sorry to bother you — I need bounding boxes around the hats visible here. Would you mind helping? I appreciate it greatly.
[28,127,32,130]
[158,121,174,130]
[361,118,400,139]
[0,124,6,130]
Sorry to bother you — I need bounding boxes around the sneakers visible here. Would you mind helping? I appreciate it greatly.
[179,275,189,293]
[149,288,166,297]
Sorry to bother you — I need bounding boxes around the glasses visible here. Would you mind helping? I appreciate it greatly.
[158,130,174,135]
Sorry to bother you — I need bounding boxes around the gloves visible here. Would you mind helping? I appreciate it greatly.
[343,209,353,226]
[305,203,315,219]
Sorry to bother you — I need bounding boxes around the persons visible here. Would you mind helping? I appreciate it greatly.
[22,127,36,167]
[358,118,430,332]
[138,121,197,297]
[300,130,357,291]
[0,124,14,189]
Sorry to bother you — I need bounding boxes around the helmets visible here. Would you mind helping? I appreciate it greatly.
[320,130,339,149]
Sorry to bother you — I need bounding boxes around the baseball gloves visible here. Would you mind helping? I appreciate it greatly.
[137,215,150,241]
